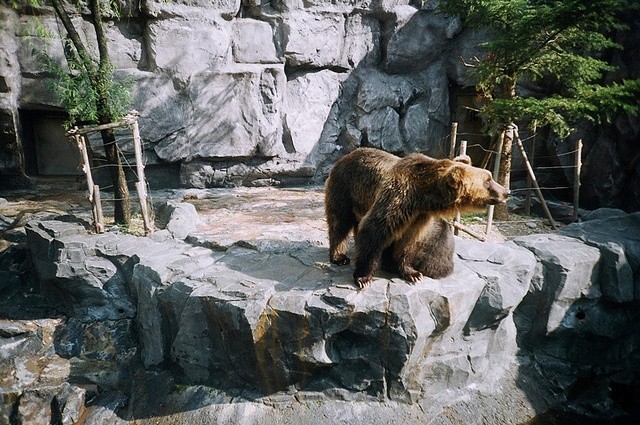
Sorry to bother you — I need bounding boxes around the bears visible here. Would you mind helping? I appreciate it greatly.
[324,147,512,289]
[405,217,455,279]
